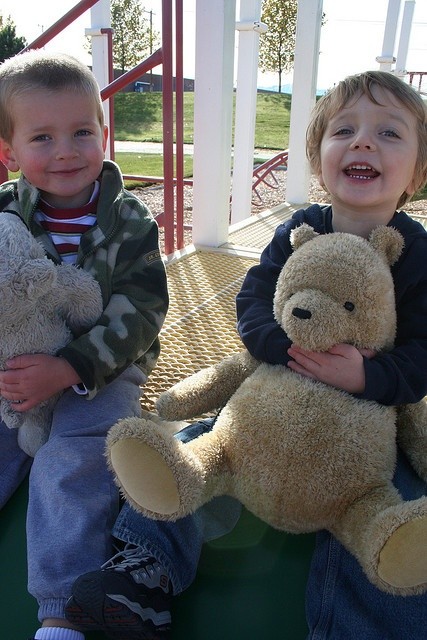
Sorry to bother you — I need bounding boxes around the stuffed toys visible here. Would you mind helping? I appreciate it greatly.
[105,224,427,597]
[1,214,103,458]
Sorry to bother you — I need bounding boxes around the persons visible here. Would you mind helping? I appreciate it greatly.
[0,49,168,639]
[57,69,427,640]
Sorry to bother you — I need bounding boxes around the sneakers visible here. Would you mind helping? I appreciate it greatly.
[65,543,172,639]
